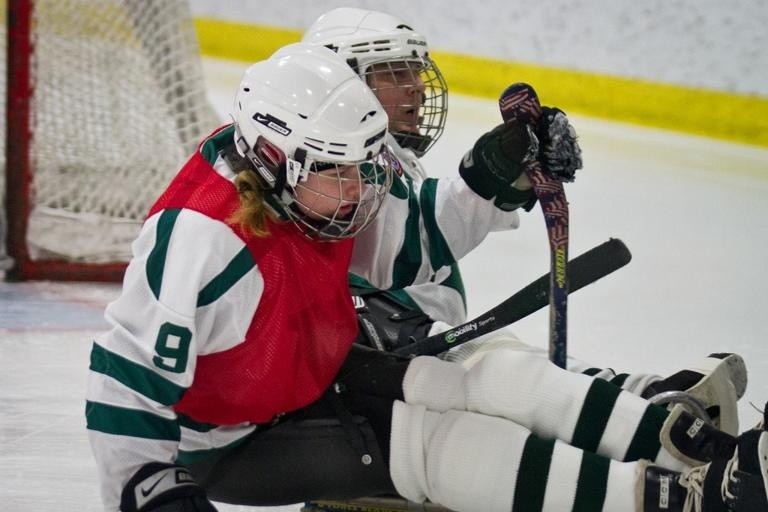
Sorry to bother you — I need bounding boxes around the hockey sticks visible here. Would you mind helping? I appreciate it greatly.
[397,237,631,357]
[498,82,571,368]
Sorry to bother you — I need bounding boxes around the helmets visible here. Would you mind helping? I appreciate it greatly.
[231,42,388,215]
[301,6,430,98]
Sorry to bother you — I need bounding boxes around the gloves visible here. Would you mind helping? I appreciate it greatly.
[458,107,583,216]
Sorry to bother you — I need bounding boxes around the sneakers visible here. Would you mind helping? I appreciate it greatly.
[634,350,767,511]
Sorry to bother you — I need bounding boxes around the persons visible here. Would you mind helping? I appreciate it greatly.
[84,40,768,512]
[301,5,750,501]
